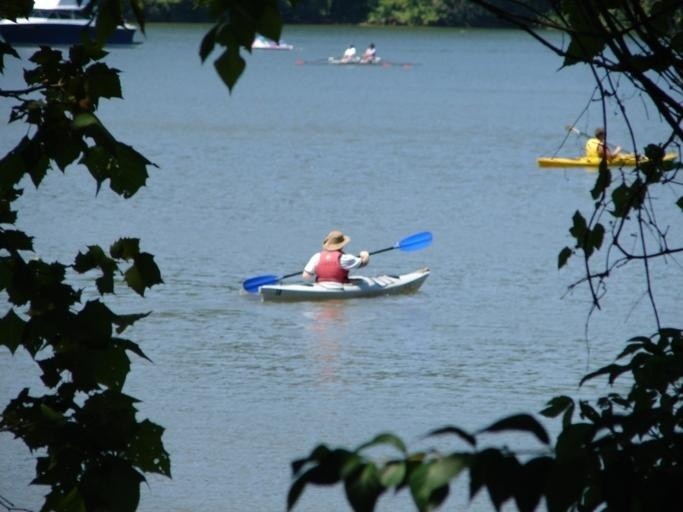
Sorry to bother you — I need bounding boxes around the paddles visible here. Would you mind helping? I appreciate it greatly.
[242,232,433,294]
[565,125,633,154]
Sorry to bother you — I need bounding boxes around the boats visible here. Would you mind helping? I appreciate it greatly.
[259,267,430,304]
[1,4,136,43]
[252,40,292,50]
[538,154,676,168]
[328,56,380,64]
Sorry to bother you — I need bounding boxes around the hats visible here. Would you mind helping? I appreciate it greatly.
[322,231,350,251]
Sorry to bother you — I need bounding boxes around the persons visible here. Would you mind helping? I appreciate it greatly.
[341,45,356,63]
[362,43,376,64]
[303,231,369,286]
[585,128,621,161]
